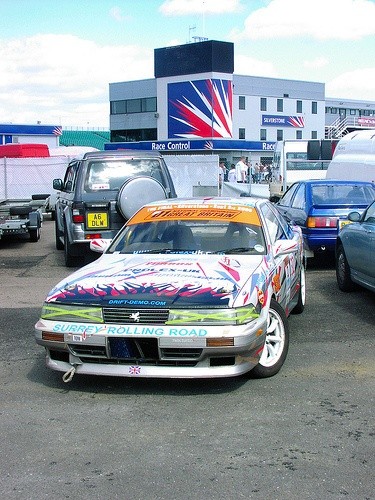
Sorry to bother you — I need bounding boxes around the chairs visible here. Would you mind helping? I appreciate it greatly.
[347,189,364,197]
[155,221,260,253]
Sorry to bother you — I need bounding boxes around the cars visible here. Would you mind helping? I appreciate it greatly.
[335,198,375,294]
[268,179,375,268]
[34,196,307,383]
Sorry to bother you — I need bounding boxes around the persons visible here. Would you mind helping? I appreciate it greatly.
[224,164,236,182]
[245,162,272,184]
[219,162,226,189]
[236,156,245,183]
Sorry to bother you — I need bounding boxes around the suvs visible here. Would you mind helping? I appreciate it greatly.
[52,149,177,269]
